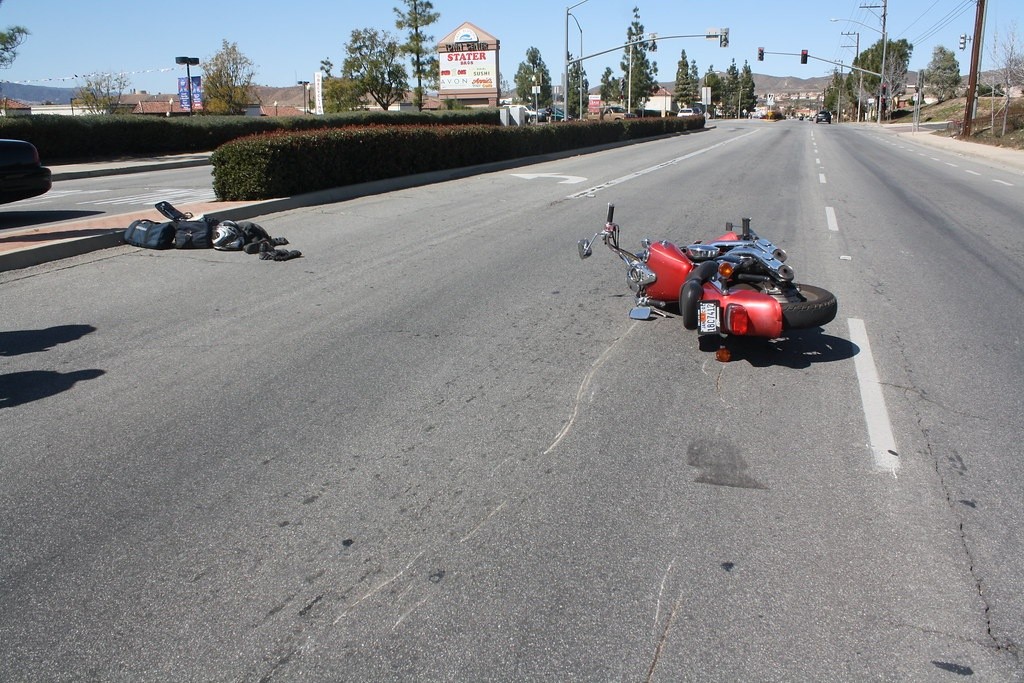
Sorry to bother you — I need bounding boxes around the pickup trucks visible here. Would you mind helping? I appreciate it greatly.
[588,107,636,121]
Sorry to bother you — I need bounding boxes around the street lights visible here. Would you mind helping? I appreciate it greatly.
[176,57,199,116]
[830,86,840,123]
[297,81,309,115]
[738,86,749,119]
[831,19,888,126]
[706,71,720,120]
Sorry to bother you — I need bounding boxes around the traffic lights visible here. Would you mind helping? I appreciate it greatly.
[959,32,965,50]
[757,47,764,61]
[801,50,808,64]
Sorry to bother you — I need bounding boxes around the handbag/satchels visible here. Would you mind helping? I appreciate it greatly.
[173,222,210,250]
[124,219,175,249]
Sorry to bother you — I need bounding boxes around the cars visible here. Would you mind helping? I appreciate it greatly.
[816,110,832,125]
[714,108,731,116]
[538,107,573,121]
[799,117,803,121]
[0,138,52,205]
[677,108,695,117]
[808,116,813,122]
[692,107,703,116]
[500,97,532,105]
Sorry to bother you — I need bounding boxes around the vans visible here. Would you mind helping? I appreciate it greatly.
[503,105,546,121]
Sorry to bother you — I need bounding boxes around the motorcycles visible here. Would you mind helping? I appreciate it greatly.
[577,202,836,367]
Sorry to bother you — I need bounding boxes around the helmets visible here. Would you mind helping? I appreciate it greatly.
[212,220,244,250]
